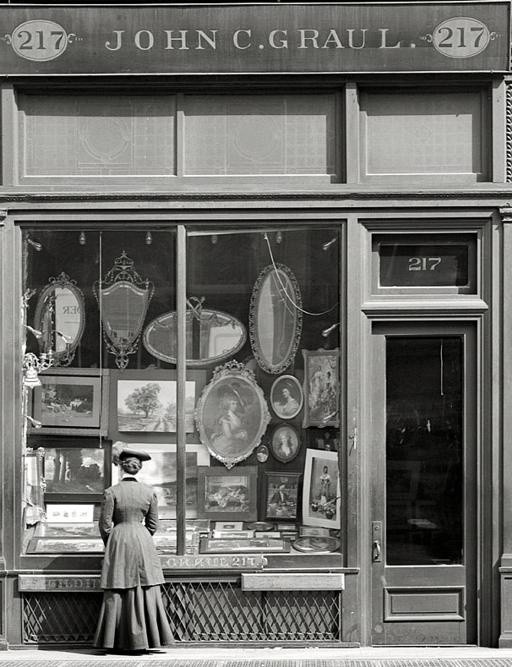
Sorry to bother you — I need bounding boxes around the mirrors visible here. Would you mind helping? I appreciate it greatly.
[142,309,247,365]
[92,248,154,369]
[249,263,304,375]
[33,271,87,365]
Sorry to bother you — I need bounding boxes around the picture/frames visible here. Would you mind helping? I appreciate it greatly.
[24,347,339,556]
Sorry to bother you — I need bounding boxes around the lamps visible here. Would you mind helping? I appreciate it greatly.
[78,230,283,247]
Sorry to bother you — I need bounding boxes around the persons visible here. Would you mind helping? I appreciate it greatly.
[312,466,331,503]
[212,392,248,454]
[277,382,299,415]
[92,448,174,651]
